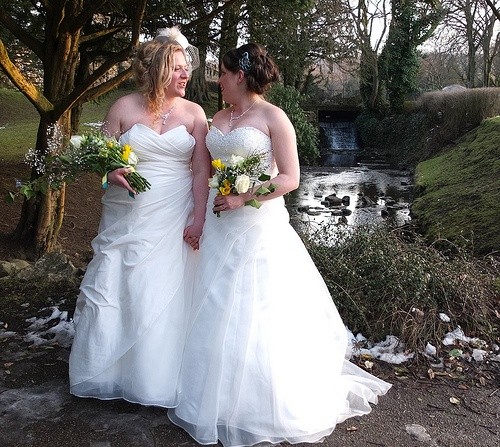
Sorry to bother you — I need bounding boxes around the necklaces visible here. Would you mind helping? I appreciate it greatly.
[156,98,178,126]
[229,98,259,126]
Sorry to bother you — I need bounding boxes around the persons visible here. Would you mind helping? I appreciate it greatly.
[69,28,209,408]
[167,42,347,447]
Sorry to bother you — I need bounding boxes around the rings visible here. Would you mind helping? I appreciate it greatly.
[222,205,225,210]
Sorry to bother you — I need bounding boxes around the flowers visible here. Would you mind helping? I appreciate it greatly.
[208,145,280,218]
[24,121,152,198]
